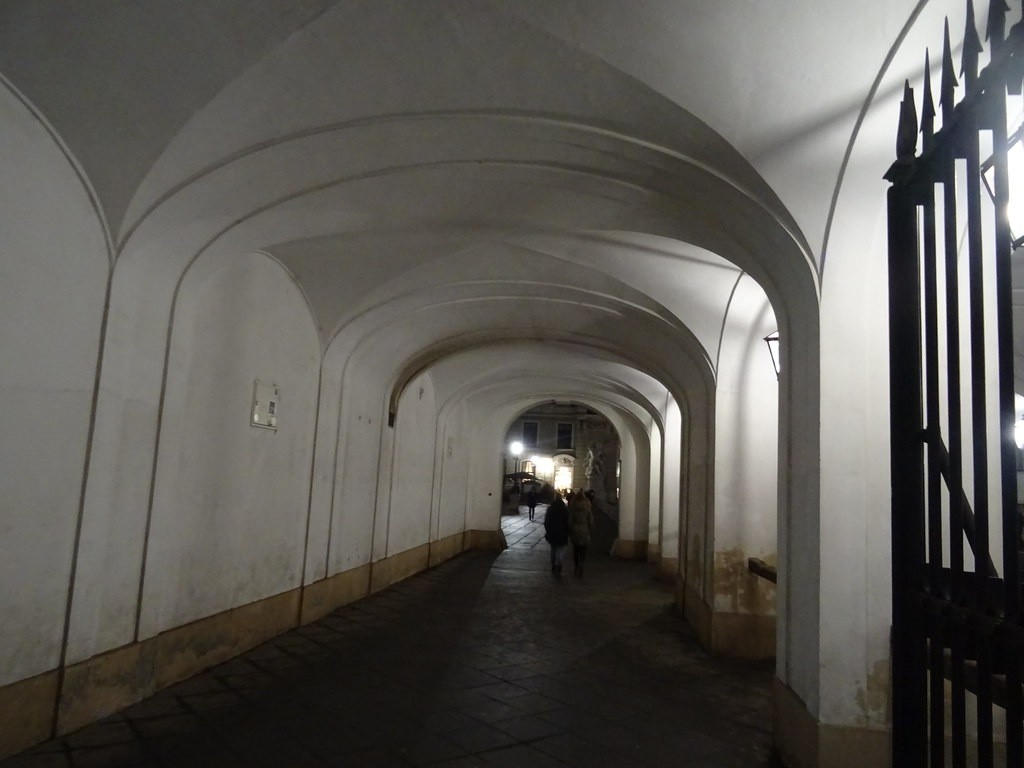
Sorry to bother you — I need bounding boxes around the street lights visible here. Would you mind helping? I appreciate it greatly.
[511,440,523,496]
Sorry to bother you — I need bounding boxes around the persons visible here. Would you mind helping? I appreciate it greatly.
[511,482,519,496]
[526,487,538,520]
[547,488,597,578]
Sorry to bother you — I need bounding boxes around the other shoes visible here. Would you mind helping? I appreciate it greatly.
[558,564,562,573]
[552,563,556,572]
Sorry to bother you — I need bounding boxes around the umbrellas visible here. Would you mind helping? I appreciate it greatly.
[504,472,539,495]
